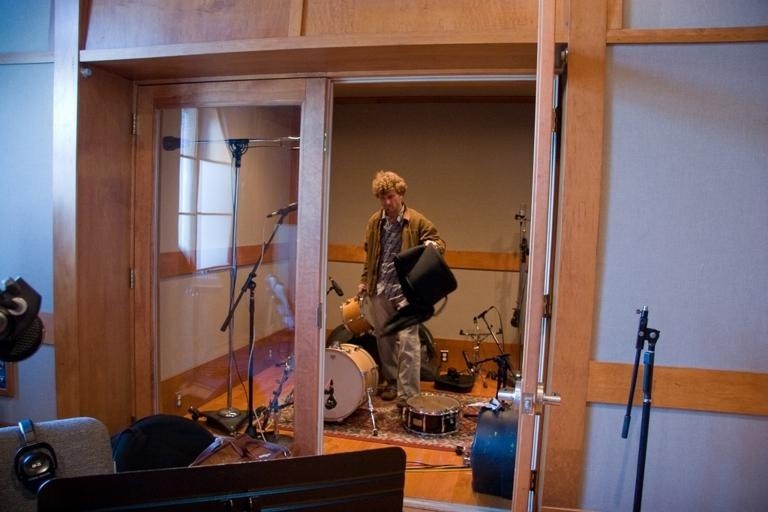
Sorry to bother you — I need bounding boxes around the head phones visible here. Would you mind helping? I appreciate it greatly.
[13,418,57,495]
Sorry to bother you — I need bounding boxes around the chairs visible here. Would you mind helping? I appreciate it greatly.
[0,418,116,512]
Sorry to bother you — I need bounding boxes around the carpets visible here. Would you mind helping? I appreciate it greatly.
[255,389,494,451]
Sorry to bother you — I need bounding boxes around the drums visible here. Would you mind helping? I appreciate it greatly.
[471,405,518,500]
[341,298,372,338]
[403,393,460,436]
[324,343,379,422]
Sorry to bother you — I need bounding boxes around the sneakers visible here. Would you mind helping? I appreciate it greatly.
[381,387,396,401]
[397,398,408,408]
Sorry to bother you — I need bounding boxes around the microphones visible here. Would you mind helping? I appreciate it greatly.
[462,351,475,374]
[329,276,344,296]
[267,201,297,218]
[478,306,493,318]
[519,203,526,226]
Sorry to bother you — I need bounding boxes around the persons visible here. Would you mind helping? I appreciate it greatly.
[355,170,446,405]
